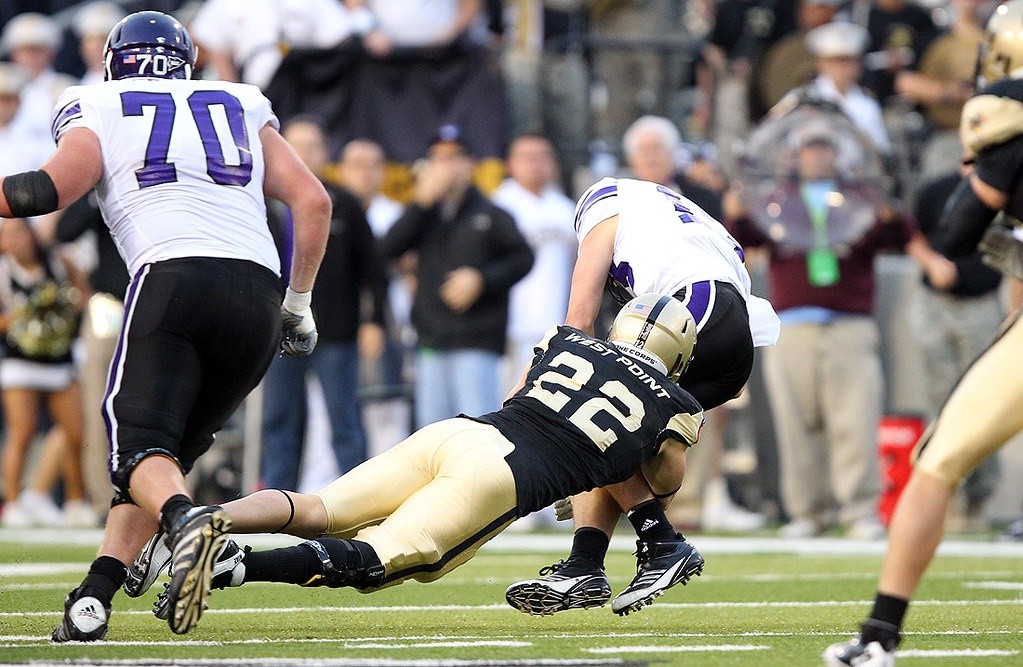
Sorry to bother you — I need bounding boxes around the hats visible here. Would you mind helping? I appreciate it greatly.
[71,3,129,37]
[0,61,37,96]
[802,20,872,59]
[3,12,64,56]
[424,124,472,153]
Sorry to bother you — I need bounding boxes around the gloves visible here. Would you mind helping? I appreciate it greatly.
[277,286,319,358]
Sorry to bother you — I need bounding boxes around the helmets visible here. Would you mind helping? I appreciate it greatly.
[975,1,1021,83]
[605,292,698,383]
[102,10,199,80]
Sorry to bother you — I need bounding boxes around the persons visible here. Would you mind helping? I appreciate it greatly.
[122,292,705,620]
[1,0,985,542]
[824,0,1023,667]
[1,11,334,642]
[504,176,781,617]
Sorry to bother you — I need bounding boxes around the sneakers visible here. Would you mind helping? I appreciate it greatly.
[820,640,899,666]
[51,588,112,640]
[612,532,706,617]
[505,559,612,617]
[122,505,250,635]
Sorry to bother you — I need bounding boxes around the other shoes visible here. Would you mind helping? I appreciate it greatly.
[701,499,765,531]
[842,514,887,541]
[0,489,103,529]
[777,515,824,538]
